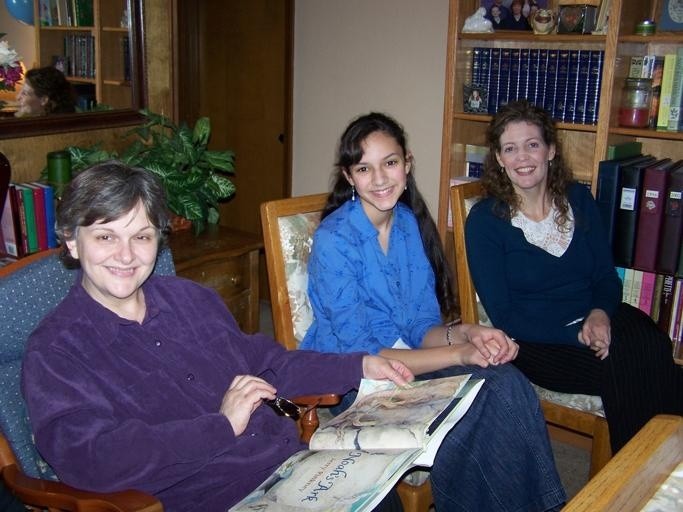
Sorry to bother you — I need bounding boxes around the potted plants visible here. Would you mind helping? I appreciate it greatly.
[39,106,238,239]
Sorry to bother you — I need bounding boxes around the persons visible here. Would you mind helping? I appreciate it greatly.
[462,98,682,460]
[491,0,539,33]
[295,109,574,512]
[19,158,415,512]
[12,63,79,120]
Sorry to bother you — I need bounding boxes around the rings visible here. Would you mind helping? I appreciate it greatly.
[508,336,516,343]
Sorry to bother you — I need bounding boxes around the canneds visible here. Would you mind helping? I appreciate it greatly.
[620,78,652,128]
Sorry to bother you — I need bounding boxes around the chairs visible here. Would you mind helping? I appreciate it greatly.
[0,239,342,512]
[255,190,437,511]
[448,178,615,479]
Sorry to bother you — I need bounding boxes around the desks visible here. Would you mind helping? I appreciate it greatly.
[558,410,683,512]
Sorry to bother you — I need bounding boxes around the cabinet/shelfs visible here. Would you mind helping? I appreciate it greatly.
[32,1,138,107]
[437,1,682,365]
[166,223,265,336]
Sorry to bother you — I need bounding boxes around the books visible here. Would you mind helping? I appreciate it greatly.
[595,52,682,370]
[357,377,486,512]
[223,373,474,512]
[453,50,606,189]
[1,173,58,263]
[35,0,97,111]
[119,36,132,84]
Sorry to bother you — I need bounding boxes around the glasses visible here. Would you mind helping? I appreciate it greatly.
[250,369,321,420]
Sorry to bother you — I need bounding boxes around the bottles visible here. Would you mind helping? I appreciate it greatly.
[46,149,72,196]
[618,75,653,130]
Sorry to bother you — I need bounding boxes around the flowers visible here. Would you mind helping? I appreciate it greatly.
[0,33,28,115]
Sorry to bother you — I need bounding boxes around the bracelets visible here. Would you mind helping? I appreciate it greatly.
[444,322,454,348]
